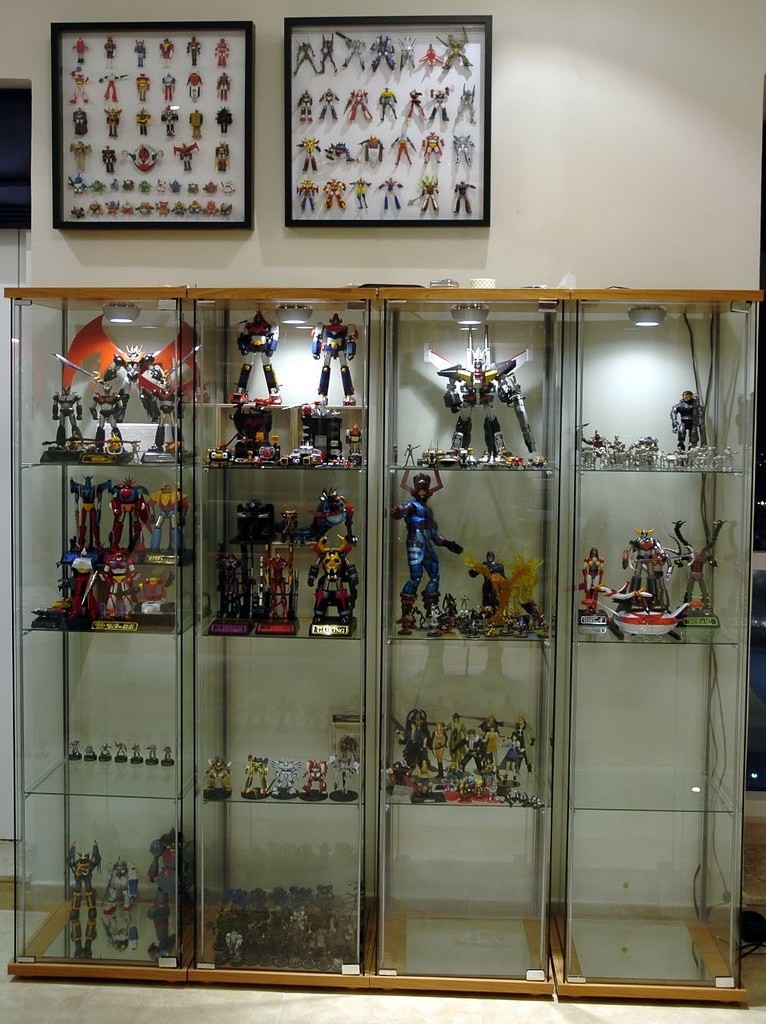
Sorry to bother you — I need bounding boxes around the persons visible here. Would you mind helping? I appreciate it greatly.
[388,710,545,807]
[577,392,739,471]
[221,490,359,624]
[392,474,551,638]
[67,829,195,917]
[67,34,236,218]
[56,475,188,620]
[582,530,720,615]
[294,33,476,214]
[207,309,364,467]
[393,346,548,469]
[204,734,359,795]
[70,917,175,960]
[52,344,177,453]
[69,739,172,761]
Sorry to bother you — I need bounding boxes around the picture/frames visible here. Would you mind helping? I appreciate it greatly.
[51,21,254,229]
[284,15,492,228]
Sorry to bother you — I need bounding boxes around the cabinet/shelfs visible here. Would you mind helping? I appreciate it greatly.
[4,288,764,1004]
